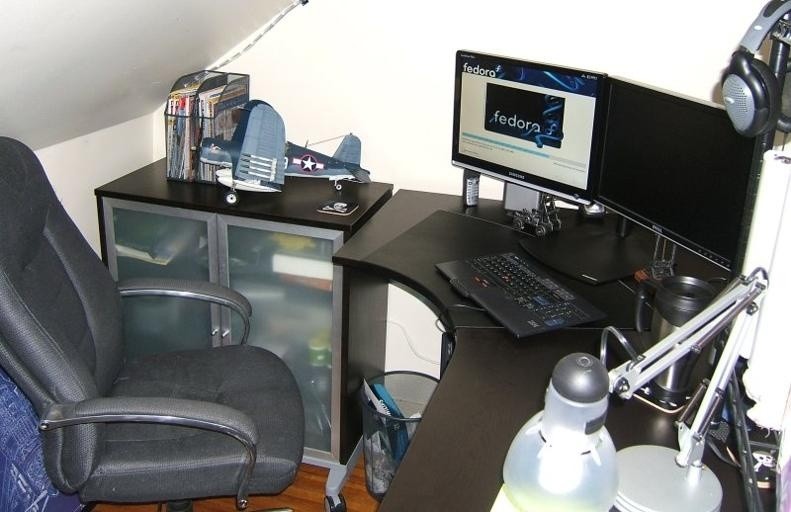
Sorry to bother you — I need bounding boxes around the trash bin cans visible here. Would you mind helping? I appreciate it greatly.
[358,371,441,503]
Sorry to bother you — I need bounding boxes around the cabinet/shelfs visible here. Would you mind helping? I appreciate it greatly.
[94,152,395,512]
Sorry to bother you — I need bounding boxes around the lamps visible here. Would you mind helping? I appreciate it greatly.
[501,265,771,512]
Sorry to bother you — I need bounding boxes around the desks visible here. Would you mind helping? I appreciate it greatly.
[332,185,784,512]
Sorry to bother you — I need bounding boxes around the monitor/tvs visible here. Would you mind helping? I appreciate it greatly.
[592,74,764,312]
[452,51,608,213]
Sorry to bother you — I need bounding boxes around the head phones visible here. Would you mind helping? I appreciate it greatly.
[720,0,791,138]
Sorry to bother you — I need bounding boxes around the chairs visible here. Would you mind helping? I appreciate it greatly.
[2,133,306,512]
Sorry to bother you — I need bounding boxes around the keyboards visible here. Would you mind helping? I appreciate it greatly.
[435,252,609,341]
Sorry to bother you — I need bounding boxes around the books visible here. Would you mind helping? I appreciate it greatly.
[165,81,246,184]
[231,250,335,362]
[115,217,199,267]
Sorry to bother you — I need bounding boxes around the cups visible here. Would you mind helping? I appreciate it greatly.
[631,274,718,407]
